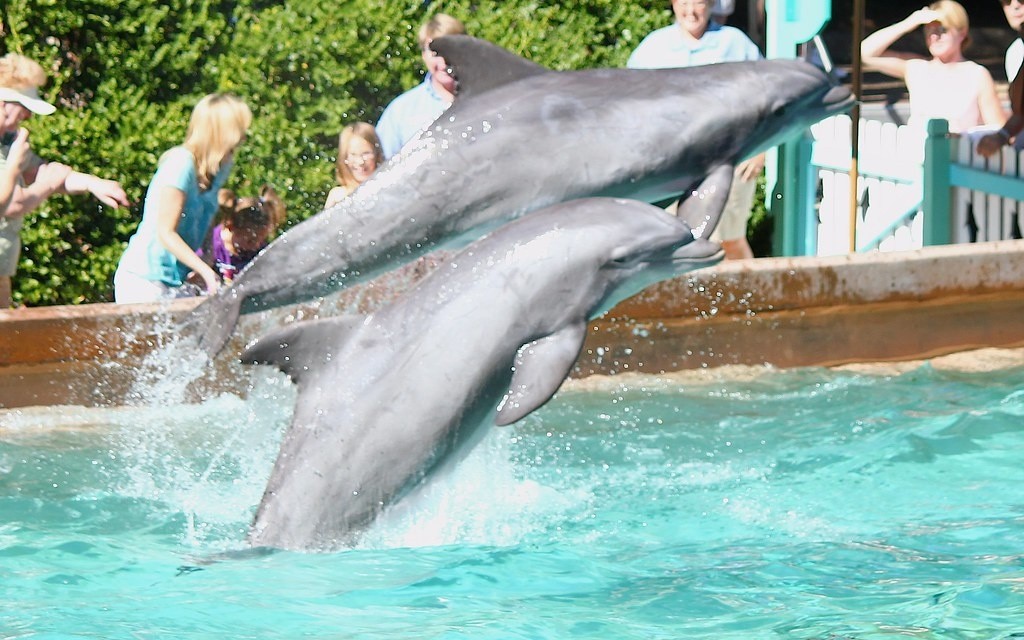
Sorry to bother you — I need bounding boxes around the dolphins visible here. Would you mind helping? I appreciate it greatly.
[177,32,859,556]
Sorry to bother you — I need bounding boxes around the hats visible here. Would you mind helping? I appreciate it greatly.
[2,78,58,117]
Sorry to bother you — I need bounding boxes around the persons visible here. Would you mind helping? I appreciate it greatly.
[0,53,140,309]
[324,14,473,210]
[860,0,1024,156]
[626,0,767,260]
[114,92,286,304]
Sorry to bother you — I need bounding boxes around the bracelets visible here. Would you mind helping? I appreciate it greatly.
[999,129,1010,140]
